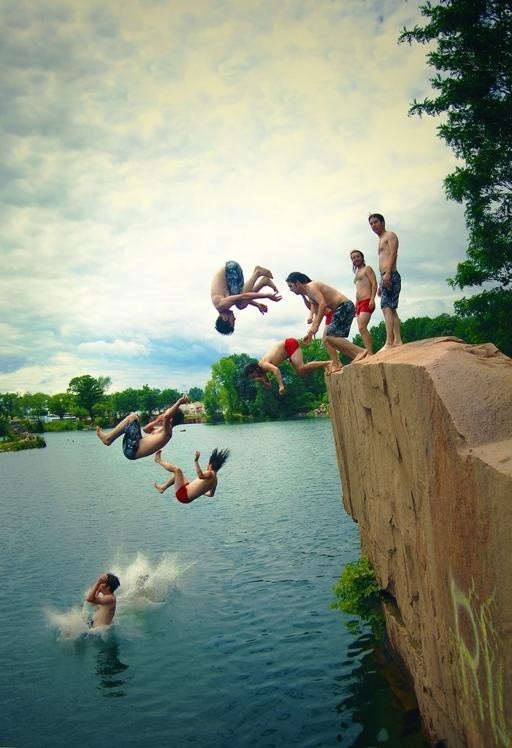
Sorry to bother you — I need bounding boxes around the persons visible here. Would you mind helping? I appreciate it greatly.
[152,447,233,504]
[242,337,335,397]
[85,574,120,628]
[208,260,283,337]
[368,213,402,355]
[287,248,378,377]
[91,393,188,459]
